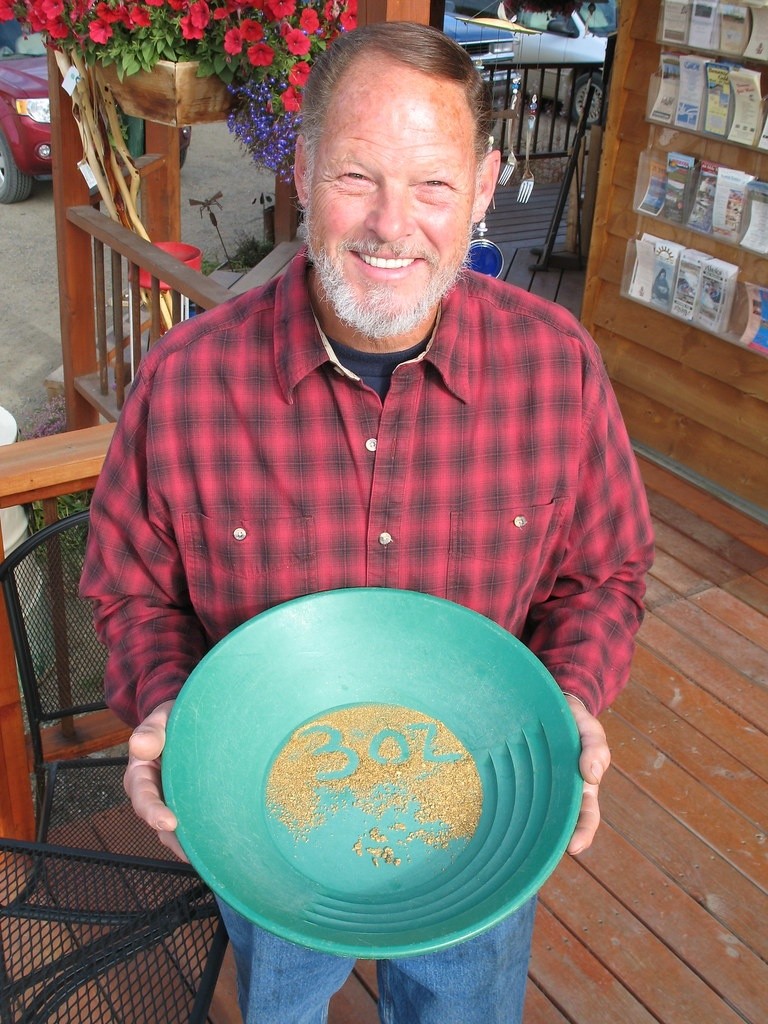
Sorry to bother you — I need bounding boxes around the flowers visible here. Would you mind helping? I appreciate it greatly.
[2,0,359,183]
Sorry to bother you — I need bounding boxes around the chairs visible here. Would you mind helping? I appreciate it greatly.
[0,512,235,926]
[2,835,231,1024]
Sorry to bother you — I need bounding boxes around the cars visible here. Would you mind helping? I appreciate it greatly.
[512,0,618,129]
[0,17,191,205]
[442,1,519,129]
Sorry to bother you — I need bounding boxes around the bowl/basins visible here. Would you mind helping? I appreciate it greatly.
[158,585,588,961]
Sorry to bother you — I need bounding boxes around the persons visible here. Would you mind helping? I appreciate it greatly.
[72,22,659,1024]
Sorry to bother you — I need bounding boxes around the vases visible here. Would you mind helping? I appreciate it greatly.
[96,52,243,127]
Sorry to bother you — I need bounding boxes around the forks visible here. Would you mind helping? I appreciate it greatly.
[497,88,519,186]
[517,94,537,204]
